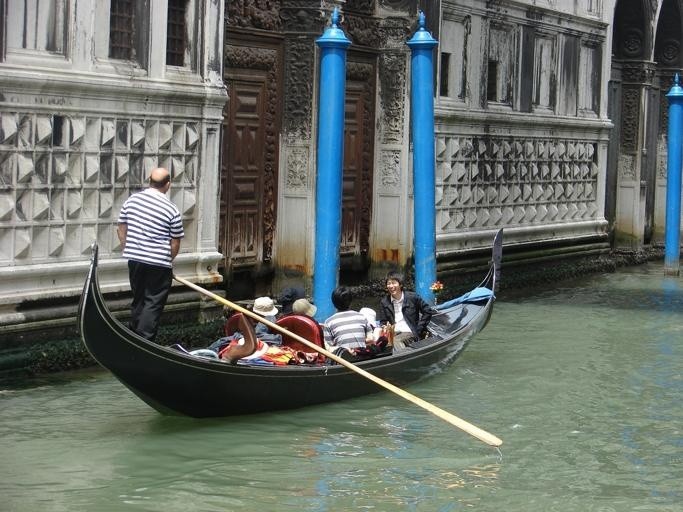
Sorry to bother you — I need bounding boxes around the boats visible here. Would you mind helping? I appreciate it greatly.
[73,227,506,420]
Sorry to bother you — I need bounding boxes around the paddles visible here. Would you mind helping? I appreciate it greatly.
[173,272,503,446]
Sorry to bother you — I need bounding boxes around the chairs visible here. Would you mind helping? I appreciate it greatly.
[270,315,326,364]
[225,313,240,336]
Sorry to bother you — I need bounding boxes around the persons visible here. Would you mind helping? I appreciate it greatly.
[116,166,185,344]
[253,274,432,366]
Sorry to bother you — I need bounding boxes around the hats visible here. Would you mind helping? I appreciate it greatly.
[252,296,279,317]
[292,298,317,318]
[359,308,377,328]
